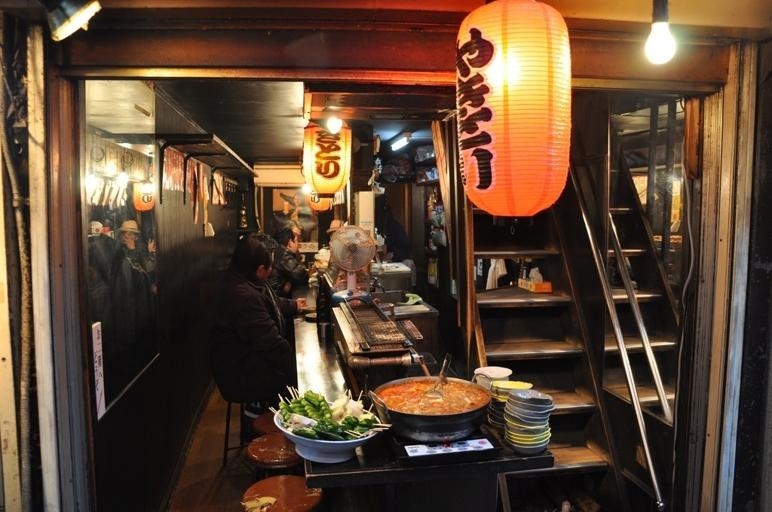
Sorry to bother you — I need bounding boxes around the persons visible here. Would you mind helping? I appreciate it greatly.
[88,217,161,316]
[199,217,313,444]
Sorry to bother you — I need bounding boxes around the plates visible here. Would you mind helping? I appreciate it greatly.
[305,313,317,318]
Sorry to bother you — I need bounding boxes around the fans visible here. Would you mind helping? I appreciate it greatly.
[329,225,377,301]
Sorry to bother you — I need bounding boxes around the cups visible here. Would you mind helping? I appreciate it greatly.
[318,322,331,338]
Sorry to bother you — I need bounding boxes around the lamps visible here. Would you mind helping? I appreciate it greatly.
[387,130,412,151]
[37,0,102,43]
[309,93,345,134]
[643,0,677,66]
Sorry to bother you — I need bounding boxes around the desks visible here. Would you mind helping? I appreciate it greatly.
[304,431,555,512]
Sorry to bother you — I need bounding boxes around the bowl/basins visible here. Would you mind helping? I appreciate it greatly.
[301,306,316,315]
[273,402,382,463]
[474,366,554,454]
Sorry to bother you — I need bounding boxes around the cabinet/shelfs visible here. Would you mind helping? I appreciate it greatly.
[408,140,450,352]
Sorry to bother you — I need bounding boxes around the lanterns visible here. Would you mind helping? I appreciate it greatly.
[306,188,334,213]
[456,0,571,219]
[302,119,353,200]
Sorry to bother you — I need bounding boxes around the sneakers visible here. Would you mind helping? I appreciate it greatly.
[244,399,272,418]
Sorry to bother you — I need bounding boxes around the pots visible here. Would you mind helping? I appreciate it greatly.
[368,375,492,444]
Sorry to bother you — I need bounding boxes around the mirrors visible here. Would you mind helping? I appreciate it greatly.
[74,78,165,422]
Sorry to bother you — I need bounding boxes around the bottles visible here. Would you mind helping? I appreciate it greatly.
[316,276,330,327]
[426,192,437,217]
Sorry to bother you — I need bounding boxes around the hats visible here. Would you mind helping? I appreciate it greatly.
[117,220,141,234]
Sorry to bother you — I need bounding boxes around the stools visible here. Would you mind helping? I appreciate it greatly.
[241,474,323,512]
[222,396,268,466]
[251,411,289,434]
[242,432,304,481]
